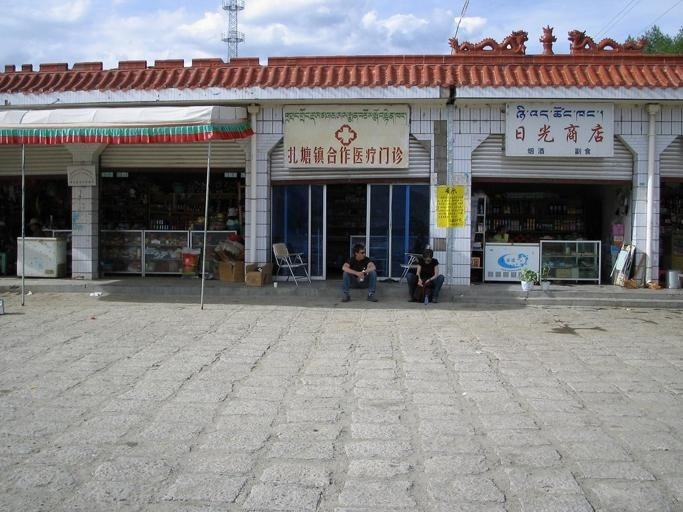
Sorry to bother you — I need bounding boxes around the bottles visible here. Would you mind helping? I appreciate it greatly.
[424,295,429,305]
[478,201,577,231]
[153,219,168,230]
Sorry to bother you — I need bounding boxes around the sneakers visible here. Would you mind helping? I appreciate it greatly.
[432,297,437,303]
[341,296,349,302]
[409,295,413,301]
[368,294,377,301]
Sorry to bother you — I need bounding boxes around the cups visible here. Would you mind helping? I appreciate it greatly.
[274,282,278,288]
[360,277,364,282]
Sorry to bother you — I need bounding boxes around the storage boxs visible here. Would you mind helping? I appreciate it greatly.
[246,265,265,287]
[219,261,244,282]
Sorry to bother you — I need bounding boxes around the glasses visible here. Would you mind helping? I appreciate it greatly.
[360,252,365,254]
[423,260,430,262]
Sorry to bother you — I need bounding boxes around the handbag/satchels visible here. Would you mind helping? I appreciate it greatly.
[413,279,432,301]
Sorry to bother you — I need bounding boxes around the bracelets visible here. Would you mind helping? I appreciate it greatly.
[429,277,431,281]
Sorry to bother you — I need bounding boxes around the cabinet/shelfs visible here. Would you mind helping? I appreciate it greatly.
[351,235,390,281]
[539,239,602,285]
[41,190,239,278]
[489,198,586,234]
[471,194,490,283]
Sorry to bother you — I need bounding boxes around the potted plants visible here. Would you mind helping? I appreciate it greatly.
[520,268,537,292]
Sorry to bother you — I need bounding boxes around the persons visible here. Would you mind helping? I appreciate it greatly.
[0,216,16,276]
[492,225,513,243]
[341,243,378,302]
[406,249,445,303]
[27,218,45,237]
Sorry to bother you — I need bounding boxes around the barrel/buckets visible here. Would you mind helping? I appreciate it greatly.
[181,253,199,272]
[665,270,681,289]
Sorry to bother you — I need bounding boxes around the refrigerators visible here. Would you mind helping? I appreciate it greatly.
[16,237,67,278]
[484,242,540,282]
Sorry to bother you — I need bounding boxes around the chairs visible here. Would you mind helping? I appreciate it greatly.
[272,242,312,289]
[399,244,431,285]
